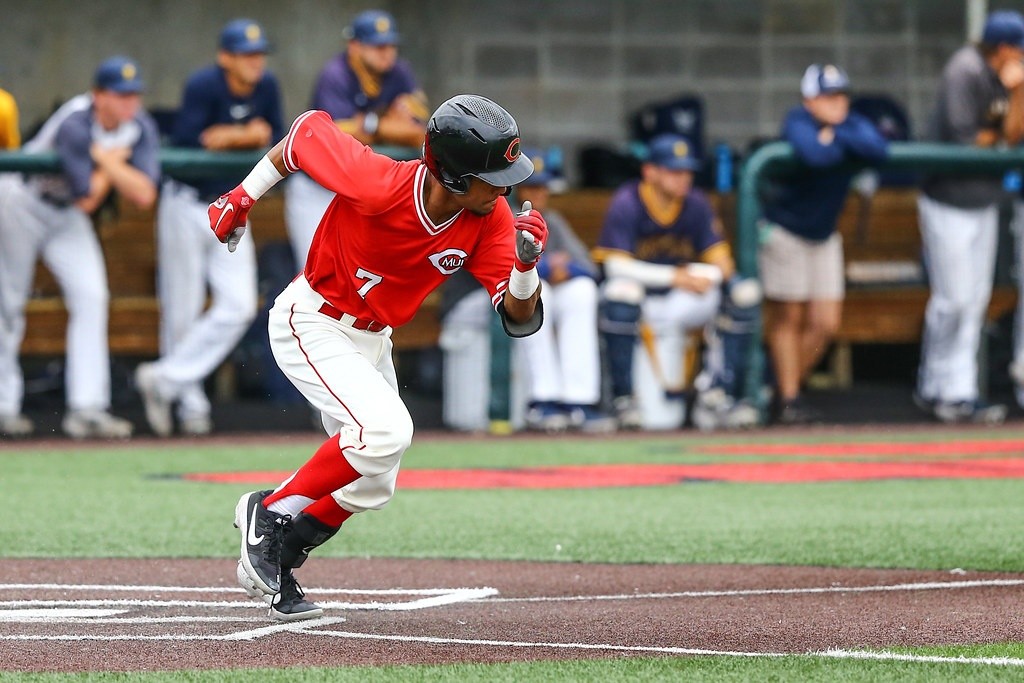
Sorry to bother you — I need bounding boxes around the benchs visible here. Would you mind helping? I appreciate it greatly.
[23,137,1015,400]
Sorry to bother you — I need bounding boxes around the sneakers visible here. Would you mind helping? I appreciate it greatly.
[234,489,296,595]
[236,547,323,621]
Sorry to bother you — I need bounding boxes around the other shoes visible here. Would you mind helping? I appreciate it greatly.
[180,411,209,438]
[915,385,1005,423]
[0,413,33,436]
[609,394,644,426]
[529,402,579,430]
[691,390,756,429]
[571,403,618,434]
[61,409,131,439]
[135,363,171,439]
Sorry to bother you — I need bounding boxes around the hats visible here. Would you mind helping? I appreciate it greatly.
[801,59,848,101]
[90,57,140,93]
[984,11,1023,47]
[649,135,696,172]
[521,148,549,186]
[219,18,274,53]
[350,10,400,47]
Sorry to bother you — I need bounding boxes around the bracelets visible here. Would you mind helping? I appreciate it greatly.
[361,110,386,145]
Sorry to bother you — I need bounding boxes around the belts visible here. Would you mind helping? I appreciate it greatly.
[292,270,386,333]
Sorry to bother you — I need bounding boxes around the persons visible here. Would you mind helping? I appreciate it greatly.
[473,153,622,436]
[290,7,431,429]
[910,7,1024,424]
[206,92,550,623]
[0,53,161,440]
[0,86,19,149]
[132,14,285,436]
[587,132,759,430]
[757,59,892,425]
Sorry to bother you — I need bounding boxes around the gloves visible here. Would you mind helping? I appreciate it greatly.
[514,201,549,272]
[206,184,256,253]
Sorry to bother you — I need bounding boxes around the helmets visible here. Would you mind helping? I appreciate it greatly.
[421,94,536,197]
